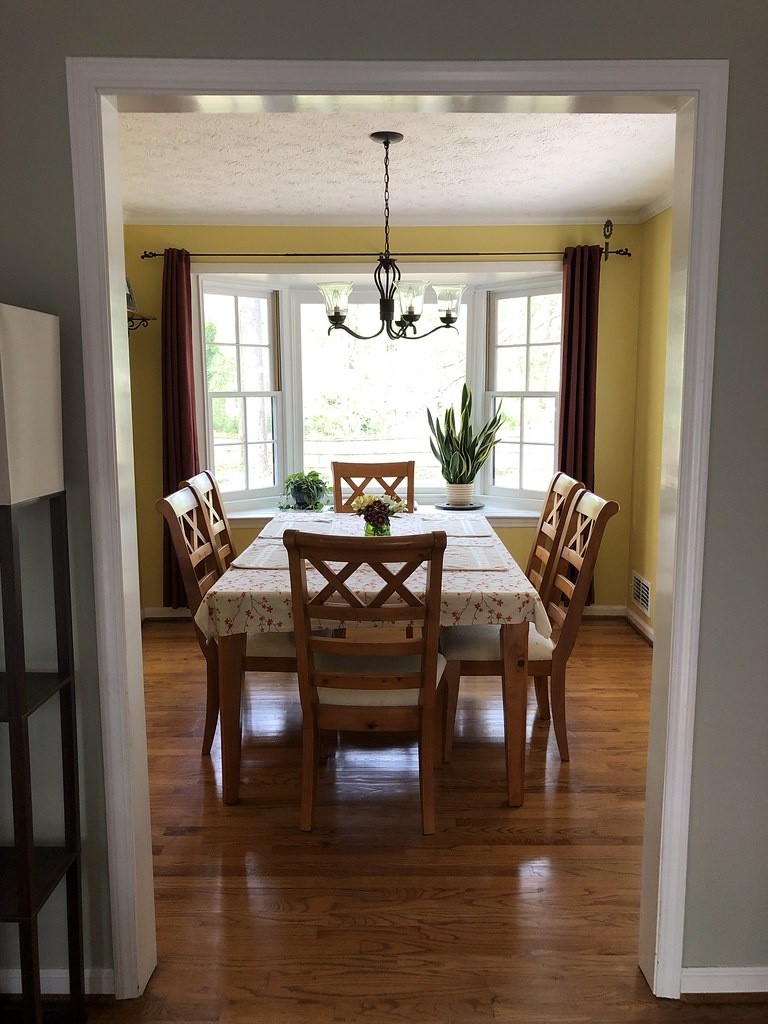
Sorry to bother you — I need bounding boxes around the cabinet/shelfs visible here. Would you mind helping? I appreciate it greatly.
[0,298,85,1024]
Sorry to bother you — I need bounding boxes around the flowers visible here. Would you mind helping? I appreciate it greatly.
[350,495,410,525]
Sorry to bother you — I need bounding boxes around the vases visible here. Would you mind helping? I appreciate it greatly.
[364,522,391,537]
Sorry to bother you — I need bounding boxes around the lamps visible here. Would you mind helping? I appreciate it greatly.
[126,273,157,342]
[316,131,468,340]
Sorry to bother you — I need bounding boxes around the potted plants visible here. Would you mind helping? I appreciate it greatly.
[278,471,335,512]
[427,383,506,505]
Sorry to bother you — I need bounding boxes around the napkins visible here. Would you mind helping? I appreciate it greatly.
[424,517,492,537]
[258,521,336,539]
[421,544,509,572]
[230,539,317,570]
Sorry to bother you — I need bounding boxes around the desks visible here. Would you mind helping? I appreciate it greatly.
[193,512,551,808]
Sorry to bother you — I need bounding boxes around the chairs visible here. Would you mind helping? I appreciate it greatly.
[523,472,585,722]
[283,528,447,836]
[440,488,620,762]
[154,484,338,757]
[179,469,347,685]
[331,460,415,513]
